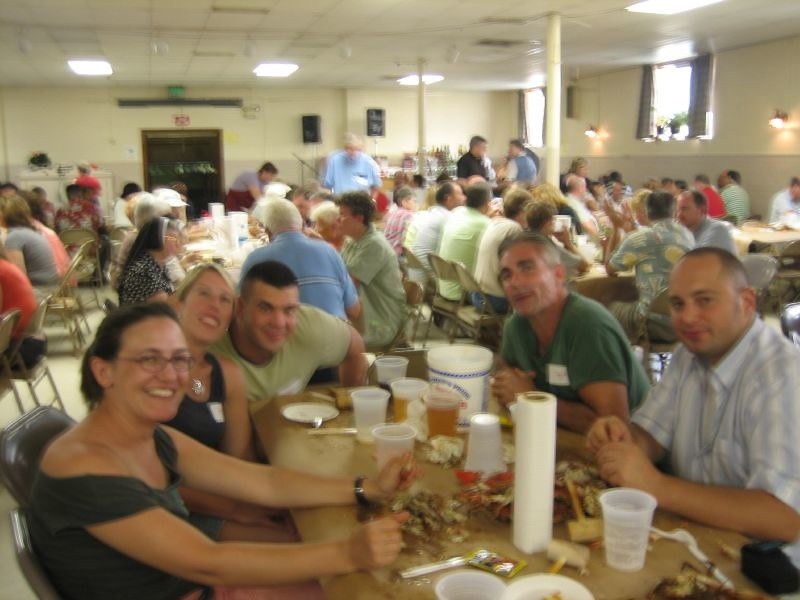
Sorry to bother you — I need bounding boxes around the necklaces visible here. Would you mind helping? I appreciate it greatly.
[192,378,206,396]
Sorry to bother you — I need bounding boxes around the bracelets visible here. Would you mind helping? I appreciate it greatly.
[355,478,371,507]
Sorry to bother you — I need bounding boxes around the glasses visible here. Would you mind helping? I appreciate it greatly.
[103,354,196,373]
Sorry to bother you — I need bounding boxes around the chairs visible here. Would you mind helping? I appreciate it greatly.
[0,225,800,600]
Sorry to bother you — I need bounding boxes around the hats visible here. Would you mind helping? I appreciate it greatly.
[121,183,140,198]
[152,188,189,208]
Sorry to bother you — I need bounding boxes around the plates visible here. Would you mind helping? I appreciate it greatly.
[503,573,594,599]
[282,402,339,422]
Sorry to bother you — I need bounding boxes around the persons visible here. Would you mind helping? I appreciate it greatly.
[0,165,110,347]
[587,246,800,600]
[210,263,367,405]
[385,136,800,344]
[27,301,417,600]
[492,231,649,432]
[114,181,188,306]
[158,260,298,544]
[230,136,406,350]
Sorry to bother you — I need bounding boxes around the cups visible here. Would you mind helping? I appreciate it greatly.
[464,414,508,480]
[435,569,508,600]
[373,423,418,474]
[599,488,658,572]
[350,356,462,443]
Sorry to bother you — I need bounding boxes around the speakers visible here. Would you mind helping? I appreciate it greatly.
[302,115,322,143]
[367,109,385,136]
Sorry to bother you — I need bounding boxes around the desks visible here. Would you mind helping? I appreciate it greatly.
[734,222,800,276]
[573,261,639,305]
[248,385,786,600]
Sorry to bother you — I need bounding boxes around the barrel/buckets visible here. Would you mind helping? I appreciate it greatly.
[425,343,494,431]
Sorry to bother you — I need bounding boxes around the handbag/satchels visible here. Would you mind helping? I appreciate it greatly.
[739,539,800,594]
